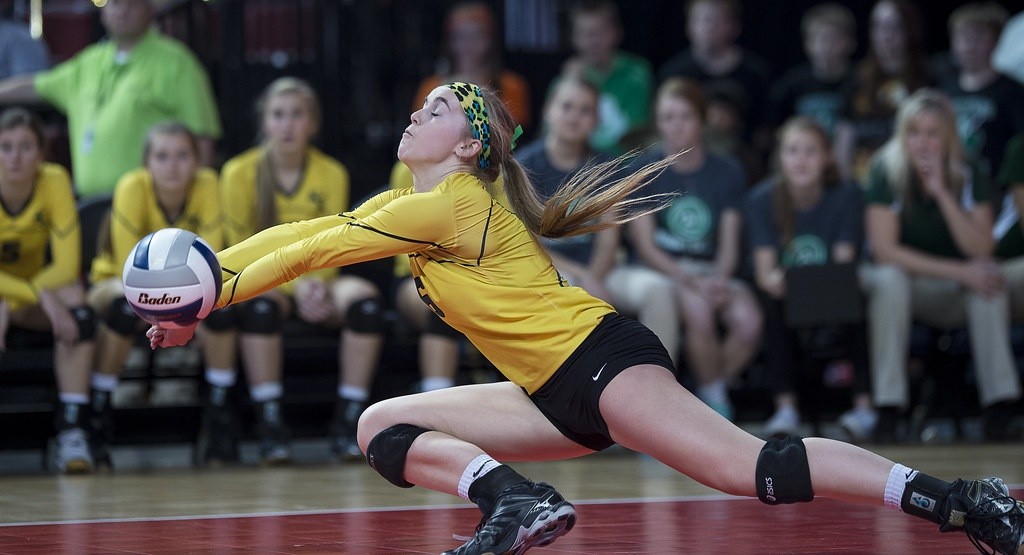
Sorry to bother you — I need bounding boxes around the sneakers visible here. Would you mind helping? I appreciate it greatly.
[939,477,1024,555]
[439,480,577,555]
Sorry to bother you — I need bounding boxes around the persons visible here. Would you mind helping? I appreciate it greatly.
[145,81,1024,555]
[0,0,1024,472]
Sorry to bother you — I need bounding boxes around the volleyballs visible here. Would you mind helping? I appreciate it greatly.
[122,228,222,329]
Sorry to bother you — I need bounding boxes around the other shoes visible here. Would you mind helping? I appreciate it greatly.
[259,414,293,464]
[54,428,93,474]
[872,408,907,449]
[841,410,875,443]
[204,422,237,469]
[763,408,797,438]
[983,411,1024,443]
[322,430,362,464]
[89,436,114,474]
